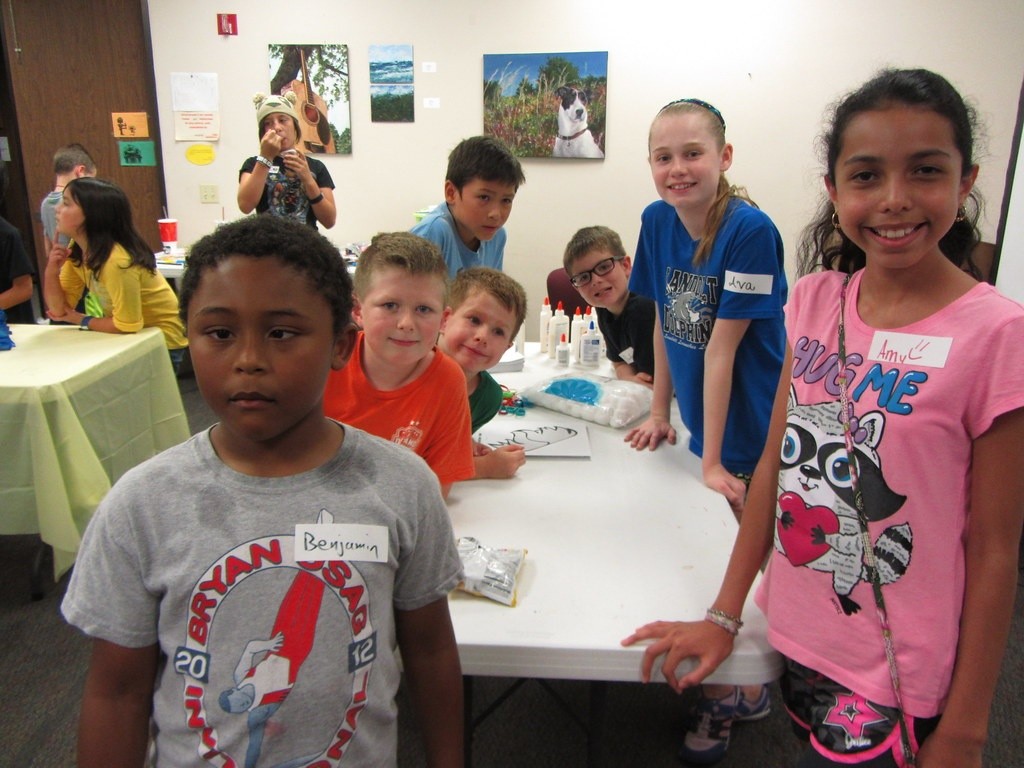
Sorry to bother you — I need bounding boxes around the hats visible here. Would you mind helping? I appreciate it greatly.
[254,90,299,125]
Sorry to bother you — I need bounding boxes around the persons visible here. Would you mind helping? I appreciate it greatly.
[317,231,475,504]
[59,215,468,768]
[236,93,337,231]
[410,135,527,283]
[619,68,1024,768]
[0,158,38,324]
[437,267,525,481]
[40,144,104,326]
[624,97,788,768]
[43,177,190,379]
[564,226,656,390]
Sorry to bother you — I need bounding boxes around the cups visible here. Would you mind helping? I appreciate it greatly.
[280,150,297,164]
[158,218,179,253]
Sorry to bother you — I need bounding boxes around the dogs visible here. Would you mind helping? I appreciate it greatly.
[552,83,605,156]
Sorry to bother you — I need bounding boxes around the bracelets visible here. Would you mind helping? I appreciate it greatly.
[256,156,271,168]
[308,193,324,204]
[81,316,93,326]
[705,609,743,634]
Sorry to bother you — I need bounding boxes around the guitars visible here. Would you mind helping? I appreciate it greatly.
[281,45,336,153]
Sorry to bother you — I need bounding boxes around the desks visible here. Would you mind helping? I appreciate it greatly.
[154,249,359,283]
[0,324,193,602]
[394,341,784,768]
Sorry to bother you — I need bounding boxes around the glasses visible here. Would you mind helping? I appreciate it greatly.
[567,256,631,287]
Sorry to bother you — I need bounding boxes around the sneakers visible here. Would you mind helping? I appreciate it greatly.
[677,690,742,767]
[689,686,773,720]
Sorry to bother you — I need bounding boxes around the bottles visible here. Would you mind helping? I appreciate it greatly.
[512,321,525,355]
[539,297,606,367]
